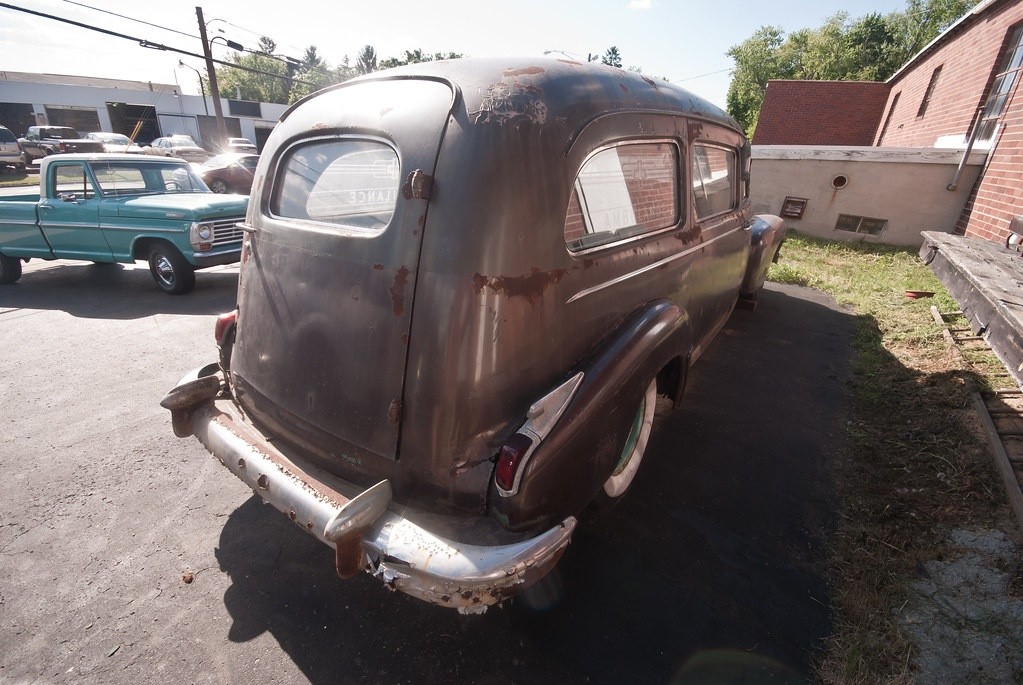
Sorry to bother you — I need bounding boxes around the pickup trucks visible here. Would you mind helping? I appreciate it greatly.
[0,152,251,297]
[17,125,104,166]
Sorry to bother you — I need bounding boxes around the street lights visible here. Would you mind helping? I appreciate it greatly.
[203,36,245,151]
[179,60,208,116]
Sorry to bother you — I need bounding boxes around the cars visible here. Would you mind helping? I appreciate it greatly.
[227,137,258,156]
[172,152,261,195]
[84,132,147,155]
[142,136,207,165]
[0,125,27,172]
[160,53,790,610]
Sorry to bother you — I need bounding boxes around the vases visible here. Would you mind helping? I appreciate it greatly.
[907,289,937,304]
